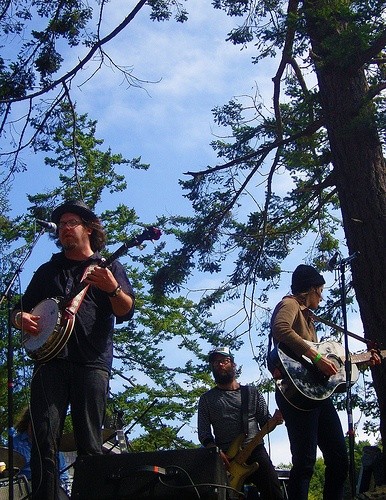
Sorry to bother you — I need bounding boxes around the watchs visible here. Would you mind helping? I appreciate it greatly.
[109,284,122,297]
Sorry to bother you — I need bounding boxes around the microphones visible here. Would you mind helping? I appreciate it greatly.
[35,219,57,233]
[327,252,339,272]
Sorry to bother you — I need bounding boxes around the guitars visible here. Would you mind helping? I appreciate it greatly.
[22,224,162,363]
[226,408,283,500]
[266,339,386,412]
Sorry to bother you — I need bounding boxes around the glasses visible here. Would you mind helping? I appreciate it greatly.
[57,219,85,229]
[212,359,231,367]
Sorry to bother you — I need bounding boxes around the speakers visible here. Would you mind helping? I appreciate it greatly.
[0,474,31,500]
[71,445,233,500]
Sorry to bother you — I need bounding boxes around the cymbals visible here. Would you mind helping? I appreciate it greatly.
[58,427,114,452]
[0,446,26,477]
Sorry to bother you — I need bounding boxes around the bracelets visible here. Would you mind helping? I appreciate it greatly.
[311,353,320,364]
[15,312,21,327]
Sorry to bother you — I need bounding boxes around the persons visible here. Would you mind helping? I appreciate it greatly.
[10,198,136,500]
[270,264,381,500]
[7,406,70,496]
[198,347,286,500]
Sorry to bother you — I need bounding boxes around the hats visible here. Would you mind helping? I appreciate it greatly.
[50,199,99,224]
[291,264,325,294]
[209,347,233,363]
